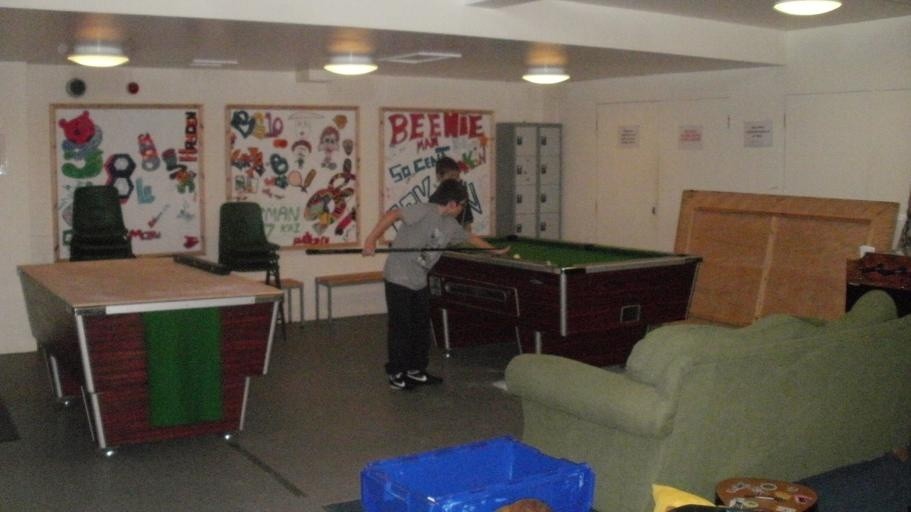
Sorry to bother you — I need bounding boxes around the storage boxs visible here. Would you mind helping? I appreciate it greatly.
[360,436,595,512]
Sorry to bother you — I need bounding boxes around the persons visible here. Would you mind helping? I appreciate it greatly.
[362,178,511,391]
[428,156,473,233]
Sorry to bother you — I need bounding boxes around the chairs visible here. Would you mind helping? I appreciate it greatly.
[70,186,135,261]
[218,201,287,339]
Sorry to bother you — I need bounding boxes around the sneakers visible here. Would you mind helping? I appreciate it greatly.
[389,372,414,391]
[405,367,443,385]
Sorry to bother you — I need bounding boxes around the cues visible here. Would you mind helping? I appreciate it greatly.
[306,249,504,254]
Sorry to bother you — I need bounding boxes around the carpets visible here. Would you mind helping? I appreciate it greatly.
[0,398,20,441]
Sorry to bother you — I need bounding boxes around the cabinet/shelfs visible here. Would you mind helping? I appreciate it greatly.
[495,122,564,244]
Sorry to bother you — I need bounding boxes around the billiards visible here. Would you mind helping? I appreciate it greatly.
[513,253,520,259]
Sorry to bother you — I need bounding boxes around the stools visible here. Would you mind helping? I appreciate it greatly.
[260,279,305,329]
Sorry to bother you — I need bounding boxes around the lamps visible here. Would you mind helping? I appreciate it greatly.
[66,14,129,69]
[322,27,379,75]
[520,43,569,86]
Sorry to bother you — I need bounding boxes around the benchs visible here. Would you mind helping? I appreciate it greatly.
[315,272,388,326]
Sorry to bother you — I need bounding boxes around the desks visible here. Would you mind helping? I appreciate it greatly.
[668,443,911,512]
[17,253,285,458]
[424,236,703,369]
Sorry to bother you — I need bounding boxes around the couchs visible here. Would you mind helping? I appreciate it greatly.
[505,289,911,512]
